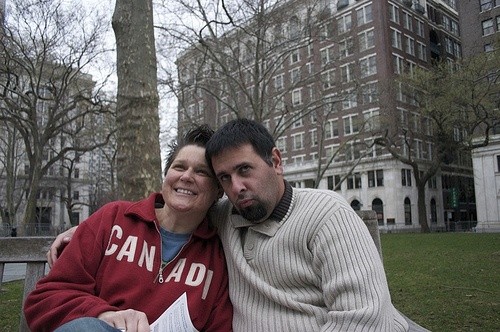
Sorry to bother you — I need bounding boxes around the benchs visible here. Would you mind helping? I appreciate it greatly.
[0,209,384,332]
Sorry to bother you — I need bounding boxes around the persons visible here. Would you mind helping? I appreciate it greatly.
[46,117,409,332]
[22,123,234,332]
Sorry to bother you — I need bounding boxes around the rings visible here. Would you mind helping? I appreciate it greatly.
[116,327,126,332]
